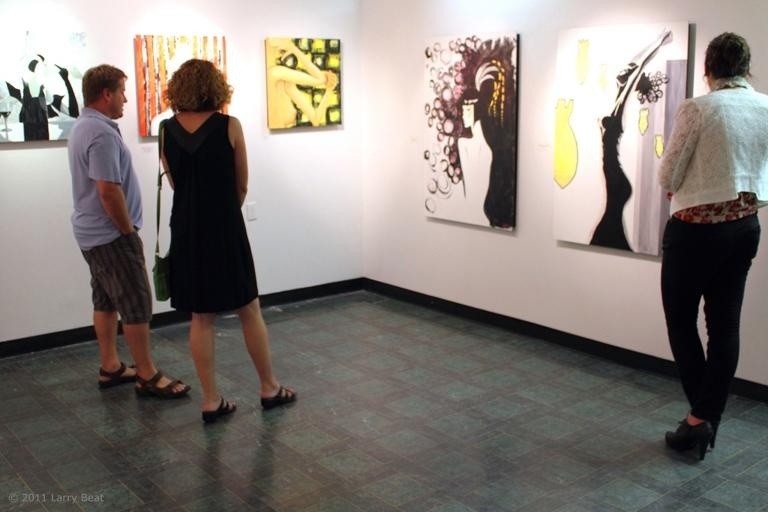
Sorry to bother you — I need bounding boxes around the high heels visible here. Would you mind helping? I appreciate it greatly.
[665,419,714,460]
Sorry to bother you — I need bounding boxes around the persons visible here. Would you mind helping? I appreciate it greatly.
[267,39,338,128]
[67,65,191,399]
[655,32,768,461]
[153,58,298,423]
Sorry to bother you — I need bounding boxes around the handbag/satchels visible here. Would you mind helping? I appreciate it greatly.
[153,253,170,300]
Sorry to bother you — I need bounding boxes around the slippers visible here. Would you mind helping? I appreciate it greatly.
[262,386,297,409]
[202,395,236,420]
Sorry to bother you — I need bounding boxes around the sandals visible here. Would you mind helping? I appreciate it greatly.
[97,365,139,387]
[135,371,191,397]
